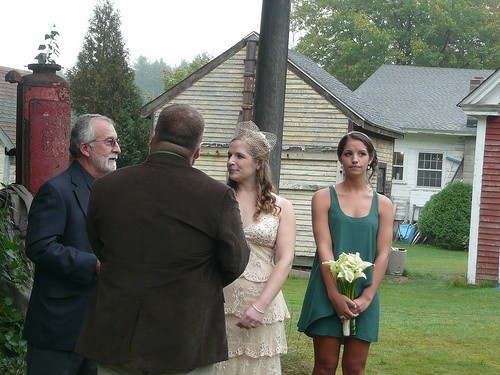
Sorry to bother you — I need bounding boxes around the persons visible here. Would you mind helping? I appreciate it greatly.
[75,104,251,375]
[297,130,395,375]
[23,113,121,375]
[187,132,296,374]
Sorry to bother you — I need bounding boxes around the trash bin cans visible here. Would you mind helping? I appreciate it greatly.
[386,246,408,277]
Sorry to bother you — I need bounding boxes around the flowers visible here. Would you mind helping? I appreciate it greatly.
[321,252,375,338]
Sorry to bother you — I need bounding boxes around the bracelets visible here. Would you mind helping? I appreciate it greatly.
[251,304,266,314]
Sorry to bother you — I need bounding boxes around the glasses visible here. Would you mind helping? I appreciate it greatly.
[88,138,121,147]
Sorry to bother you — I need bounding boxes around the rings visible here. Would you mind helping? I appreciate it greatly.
[250,322,255,328]
[340,315,344,319]
